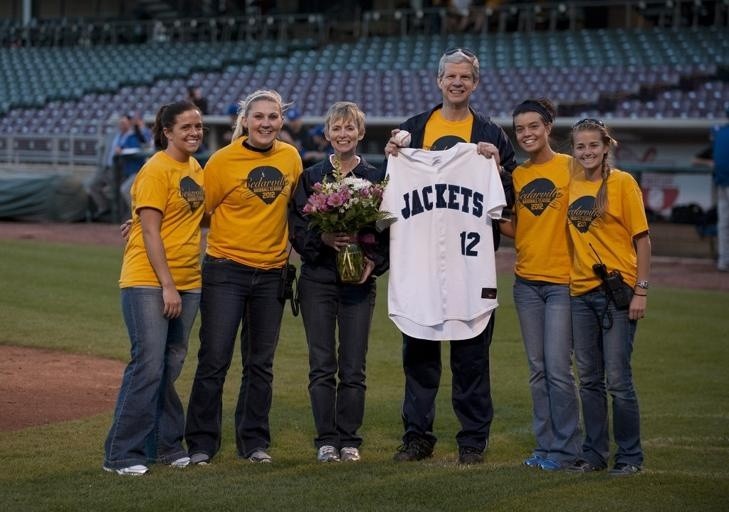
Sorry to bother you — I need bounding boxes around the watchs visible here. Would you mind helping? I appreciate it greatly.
[635,278,649,289]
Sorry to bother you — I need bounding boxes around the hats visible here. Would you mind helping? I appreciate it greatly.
[308,125,323,136]
[224,105,238,114]
[287,109,299,121]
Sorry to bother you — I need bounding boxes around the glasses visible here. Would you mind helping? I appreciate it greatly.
[574,118,604,127]
[445,46,475,58]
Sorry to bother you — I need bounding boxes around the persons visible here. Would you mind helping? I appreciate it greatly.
[710,107,728,272]
[85,87,333,221]
[382,48,516,469]
[501,96,586,475]
[100,100,206,477]
[566,116,653,475]
[396,0,568,34]
[285,99,386,463]
[117,88,304,468]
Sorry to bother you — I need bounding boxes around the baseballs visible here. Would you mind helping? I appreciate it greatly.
[395,130,412,147]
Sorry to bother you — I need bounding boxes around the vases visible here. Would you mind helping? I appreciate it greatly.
[336,243,363,282]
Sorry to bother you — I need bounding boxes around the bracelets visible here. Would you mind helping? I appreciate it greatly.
[633,292,647,297]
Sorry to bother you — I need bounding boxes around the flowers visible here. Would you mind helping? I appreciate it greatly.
[300,159,397,279]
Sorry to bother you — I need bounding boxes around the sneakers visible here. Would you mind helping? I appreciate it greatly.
[317,444,362,464]
[609,464,640,475]
[457,444,482,464]
[393,441,430,463]
[246,448,273,464]
[568,461,602,475]
[169,454,210,468]
[525,453,572,469]
[104,462,149,476]
